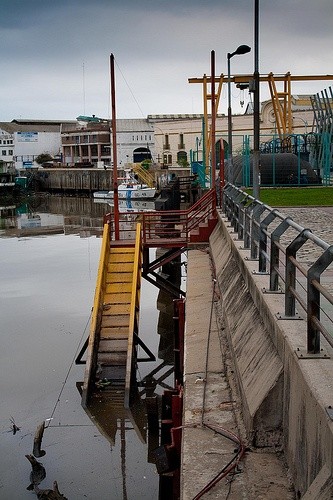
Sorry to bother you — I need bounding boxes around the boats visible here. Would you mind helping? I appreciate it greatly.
[94,198,156,221]
[93,170,157,199]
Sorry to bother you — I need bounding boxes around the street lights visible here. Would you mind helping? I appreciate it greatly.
[227,44,251,187]
